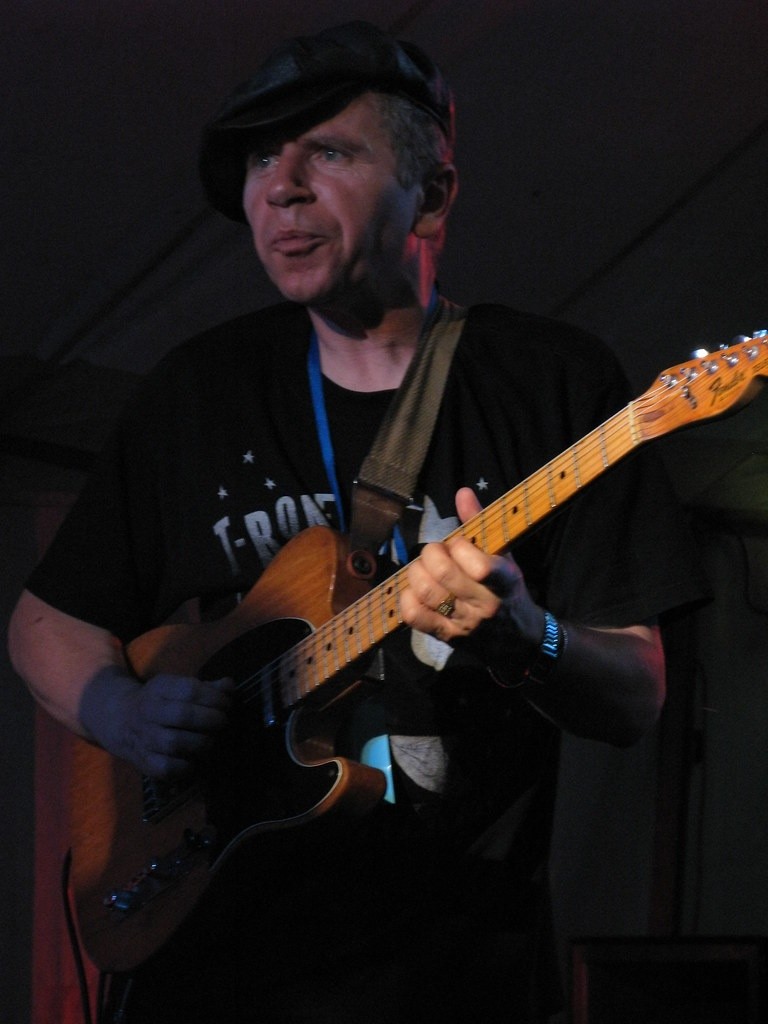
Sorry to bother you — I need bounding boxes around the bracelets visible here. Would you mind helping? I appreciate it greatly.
[558,623,568,665]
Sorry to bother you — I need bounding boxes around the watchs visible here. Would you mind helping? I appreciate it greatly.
[528,610,562,684]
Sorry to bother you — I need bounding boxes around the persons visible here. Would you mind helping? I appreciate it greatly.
[6,23,715,1024]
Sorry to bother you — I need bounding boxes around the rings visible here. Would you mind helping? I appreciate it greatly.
[437,593,457,617]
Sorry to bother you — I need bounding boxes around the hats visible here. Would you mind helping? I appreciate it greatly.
[199,17,458,154]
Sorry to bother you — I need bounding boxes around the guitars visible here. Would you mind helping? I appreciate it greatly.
[67,328,768,976]
